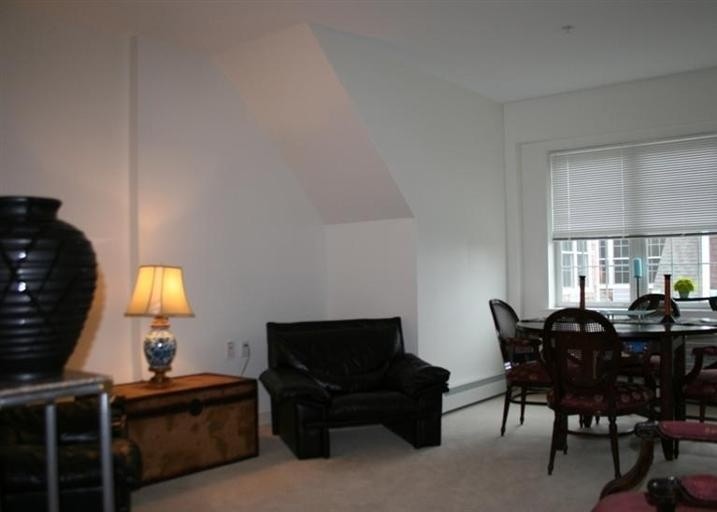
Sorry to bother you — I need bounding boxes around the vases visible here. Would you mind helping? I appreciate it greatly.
[678,290,689,298]
[0,194,99,382]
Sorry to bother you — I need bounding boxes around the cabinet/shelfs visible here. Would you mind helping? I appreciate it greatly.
[108,371,259,490]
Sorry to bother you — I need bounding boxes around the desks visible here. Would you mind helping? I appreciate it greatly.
[0,377,134,512]
[513,316,717,463]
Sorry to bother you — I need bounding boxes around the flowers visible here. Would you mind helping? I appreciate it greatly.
[674,278,694,292]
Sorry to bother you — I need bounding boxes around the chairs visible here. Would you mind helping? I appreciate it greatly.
[628,293,678,320]
[258,315,451,458]
[542,307,656,479]
[678,346,716,442]
[489,299,572,441]
[587,421,717,512]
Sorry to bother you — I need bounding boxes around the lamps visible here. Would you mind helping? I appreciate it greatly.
[123,264,194,388]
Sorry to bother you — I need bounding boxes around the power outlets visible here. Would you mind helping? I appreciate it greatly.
[239,338,251,357]
